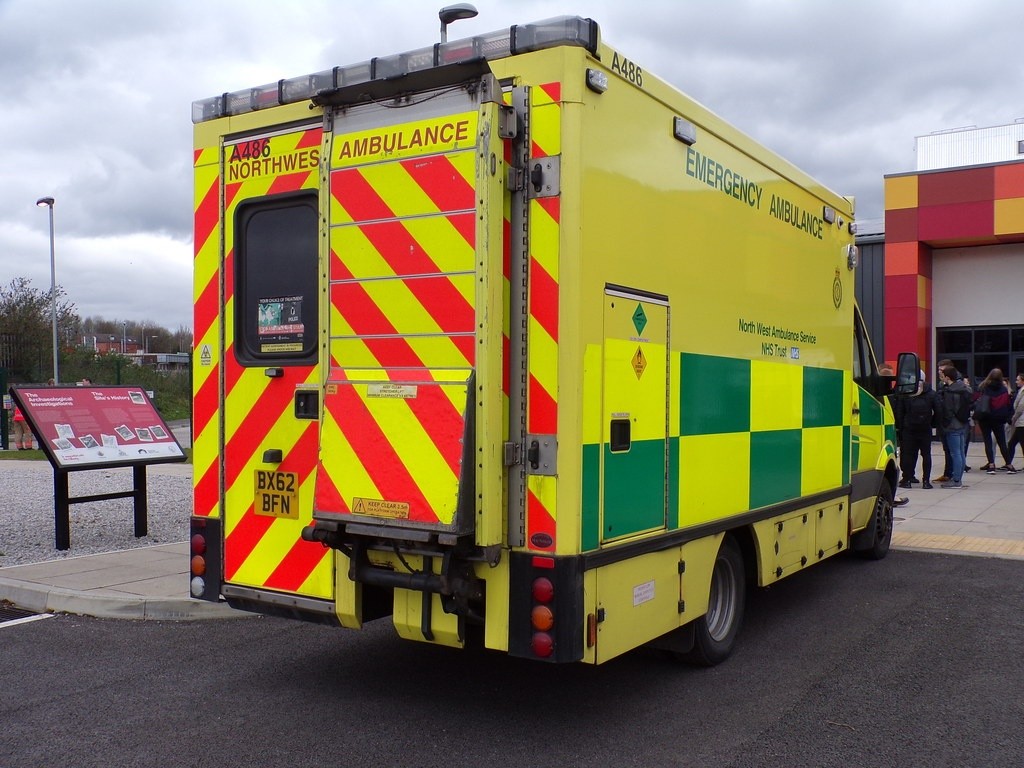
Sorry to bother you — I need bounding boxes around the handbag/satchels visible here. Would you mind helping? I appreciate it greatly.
[972,388,990,420]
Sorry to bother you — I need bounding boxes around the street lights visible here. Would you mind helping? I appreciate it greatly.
[36,197,59,387]
[438,2,478,42]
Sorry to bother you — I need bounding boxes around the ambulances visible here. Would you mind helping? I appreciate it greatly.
[190,10,921,669]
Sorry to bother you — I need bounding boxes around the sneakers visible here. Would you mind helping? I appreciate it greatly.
[1006,465,1017,474]
[986,463,996,475]
[941,479,962,488]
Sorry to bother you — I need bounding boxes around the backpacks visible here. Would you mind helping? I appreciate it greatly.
[909,392,934,430]
[953,391,972,424]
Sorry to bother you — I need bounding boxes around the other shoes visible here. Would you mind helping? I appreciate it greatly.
[932,476,950,483]
[980,463,988,470]
[899,477,919,483]
[1000,464,1006,470]
[964,465,971,473]
[923,482,933,489]
[899,480,912,488]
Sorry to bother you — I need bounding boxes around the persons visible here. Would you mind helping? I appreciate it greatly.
[82,376,93,385]
[13,405,34,450]
[878,352,1024,507]
[47,378,55,386]
